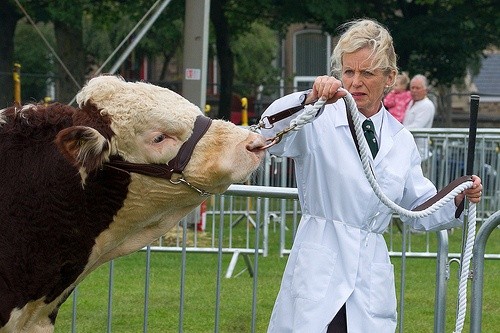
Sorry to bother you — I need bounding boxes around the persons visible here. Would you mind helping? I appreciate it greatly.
[257,19,484,333]
[396,74,435,175]
[381,72,415,127]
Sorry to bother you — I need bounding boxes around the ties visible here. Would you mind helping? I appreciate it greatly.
[361,119,378,161]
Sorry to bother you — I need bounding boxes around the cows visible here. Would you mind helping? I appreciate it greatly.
[1,75,266,332]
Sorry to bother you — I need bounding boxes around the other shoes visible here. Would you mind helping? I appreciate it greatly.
[408,228,426,234]
